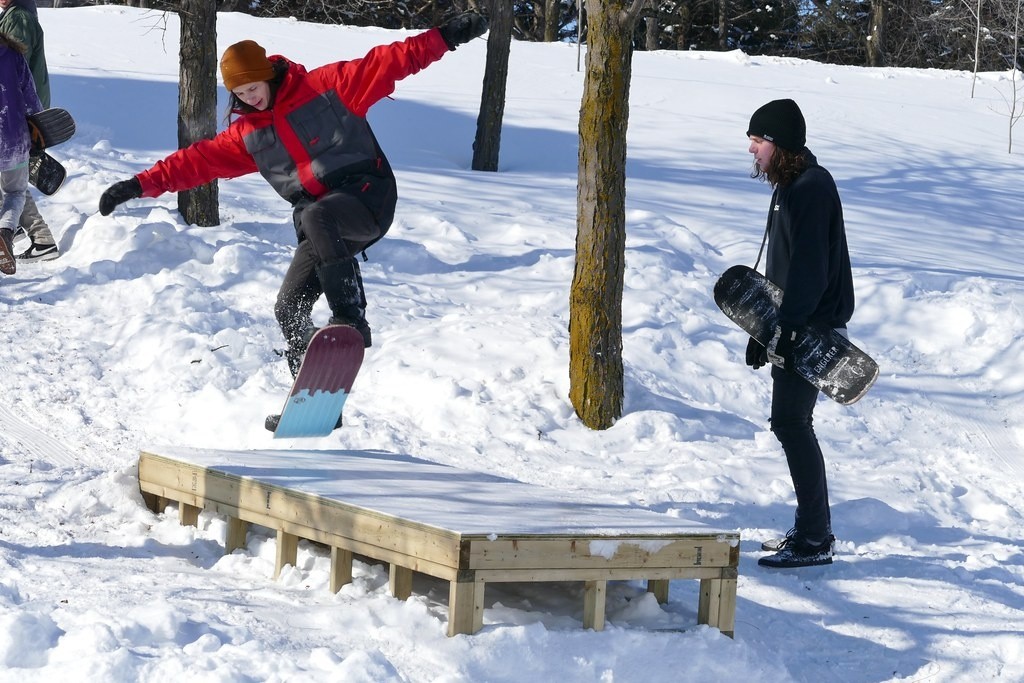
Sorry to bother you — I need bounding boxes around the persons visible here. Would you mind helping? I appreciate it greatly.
[99,7,488,432]
[0,0,59,275]
[746,99,855,568]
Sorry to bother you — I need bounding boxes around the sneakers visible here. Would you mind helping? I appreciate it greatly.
[761,534,835,556]
[11,243,60,263]
[12,226,27,245]
[758,534,832,567]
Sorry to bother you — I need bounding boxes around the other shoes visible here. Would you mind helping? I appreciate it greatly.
[0,228,17,275]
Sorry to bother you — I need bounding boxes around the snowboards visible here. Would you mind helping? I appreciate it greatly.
[713,264,879,404]
[273,321,365,441]
[28,149,69,198]
[27,107,76,151]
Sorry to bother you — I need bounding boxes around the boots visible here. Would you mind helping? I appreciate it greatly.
[265,346,342,431]
[303,259,371,346]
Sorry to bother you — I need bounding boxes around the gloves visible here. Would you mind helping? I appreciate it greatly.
[759,325,798,370]
[746,336,764,369]
[99,177,143,216]
[439,8,488,51]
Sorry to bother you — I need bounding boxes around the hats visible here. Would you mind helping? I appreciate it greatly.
[746,99,806,154]
[220,40,274,91]
